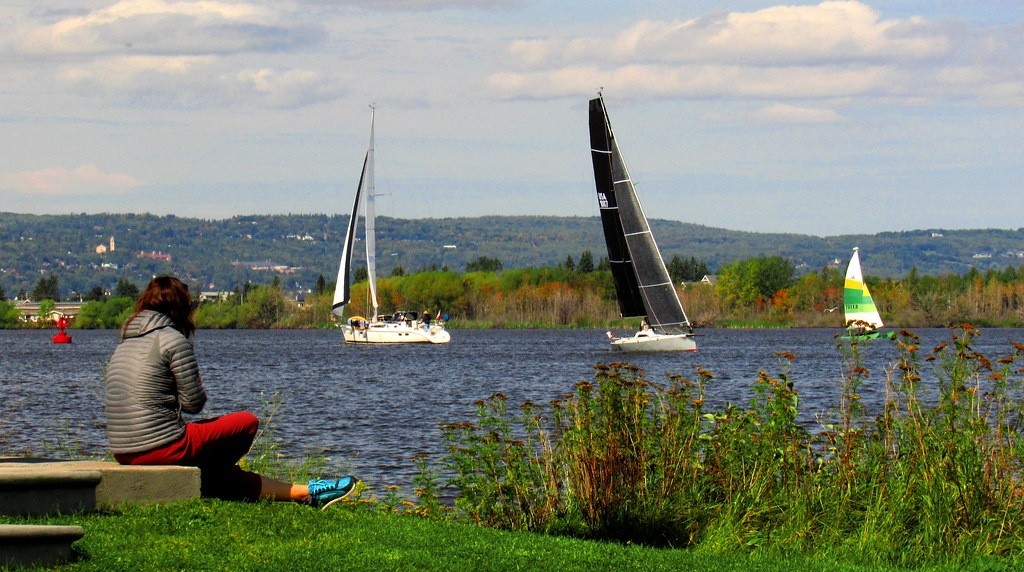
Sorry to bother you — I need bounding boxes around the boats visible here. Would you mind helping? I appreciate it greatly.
[348,316,368,333]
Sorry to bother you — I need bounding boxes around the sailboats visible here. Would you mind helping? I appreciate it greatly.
[589,86,697,354]
[331,103,451,344]
[841,247,897,341]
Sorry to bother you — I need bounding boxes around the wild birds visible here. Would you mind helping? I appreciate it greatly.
[23,298,30,305]
[821,306,840,319]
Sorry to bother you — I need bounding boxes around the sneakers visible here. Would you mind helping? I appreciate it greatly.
[307,476,355,511]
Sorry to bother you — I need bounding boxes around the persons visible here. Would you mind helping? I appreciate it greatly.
[421,310,432,330]
[104,274,358,511]
